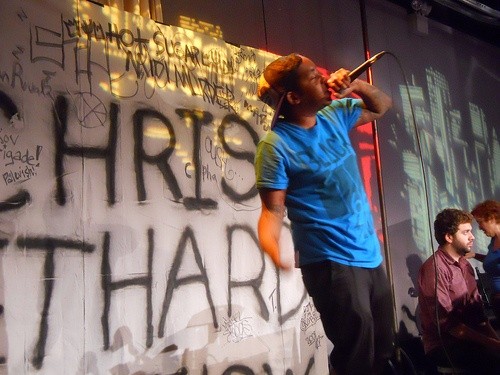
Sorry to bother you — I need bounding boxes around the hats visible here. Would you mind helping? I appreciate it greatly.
[257,54,302,130]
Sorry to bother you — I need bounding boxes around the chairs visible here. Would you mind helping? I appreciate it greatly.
[399,337,463,375]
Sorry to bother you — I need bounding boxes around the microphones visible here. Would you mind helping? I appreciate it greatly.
[348,51,386,83]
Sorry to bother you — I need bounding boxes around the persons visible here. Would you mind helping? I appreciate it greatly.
[417,209,500,374]
[255,52,394,375]
[463,199,500,325]
